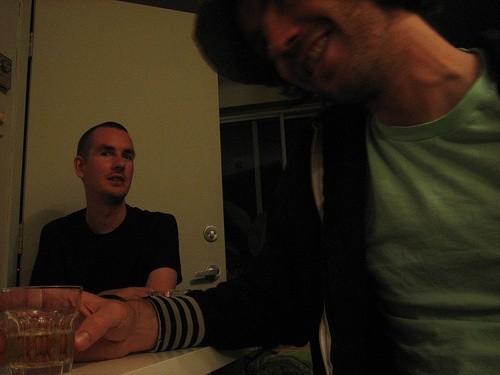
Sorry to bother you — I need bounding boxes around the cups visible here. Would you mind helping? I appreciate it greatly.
[1,286,83,375]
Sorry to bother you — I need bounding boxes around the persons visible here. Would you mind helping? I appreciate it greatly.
[28,121,183,301]
[0,0,500,375]
